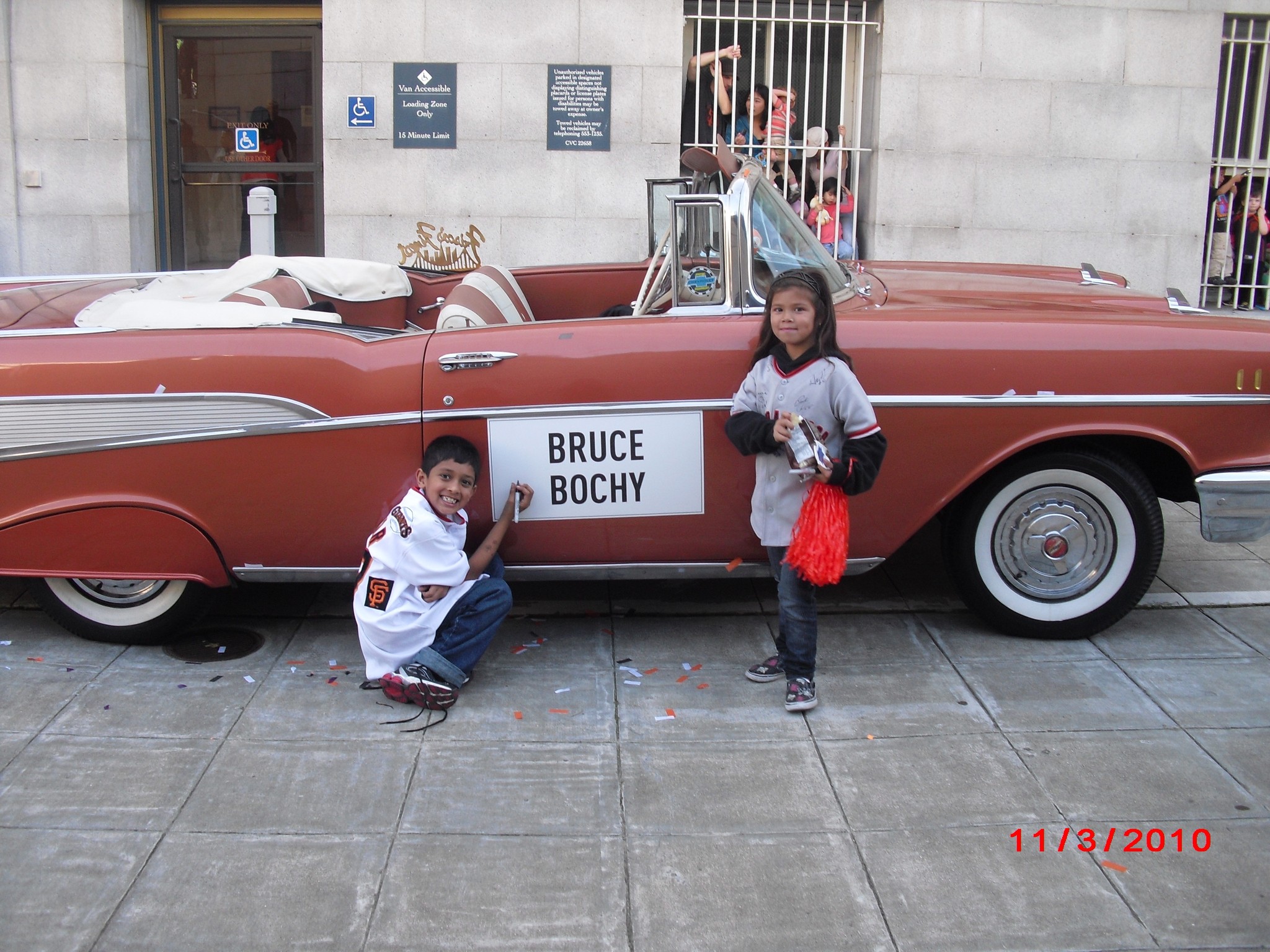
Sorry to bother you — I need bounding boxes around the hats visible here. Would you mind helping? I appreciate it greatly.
[720,56,740,81]
[801,126,828,157]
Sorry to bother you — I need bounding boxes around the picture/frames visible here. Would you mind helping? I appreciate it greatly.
[208,106,240,129]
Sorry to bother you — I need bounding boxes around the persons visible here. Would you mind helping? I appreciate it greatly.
[272,101,297,221]
[762,86,801,203]
[726,84,797,248]
[1206,161,1244,285]
[353,434,534,732]
[725,268,887,711]
[807,177,854,260]
[681,45,741,154]
[802,125,858,260]
[788,176,809,224]
[1222,182,1270,311]
[227,106,293,259]
[177,120,245,263]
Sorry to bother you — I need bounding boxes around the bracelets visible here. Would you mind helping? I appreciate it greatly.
[775,154,778,162]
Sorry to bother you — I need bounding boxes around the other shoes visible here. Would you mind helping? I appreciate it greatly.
[785,677,818,710]
[745,653,785,683]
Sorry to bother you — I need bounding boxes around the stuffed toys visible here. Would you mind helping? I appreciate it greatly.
[810,195,833,225]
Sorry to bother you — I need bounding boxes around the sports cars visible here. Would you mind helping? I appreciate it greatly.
[1,155,1269,649]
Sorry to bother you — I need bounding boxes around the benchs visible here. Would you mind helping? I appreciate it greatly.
[220,274,314,309]
[436,263,535,329]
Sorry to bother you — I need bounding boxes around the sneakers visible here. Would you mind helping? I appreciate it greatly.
[1221,298,1233,306]
[1237,301,1249,311]
[1207,276,1226,285]
[776,187,783,194]
[1223,276,1237,285]
[379,665,458,710]
[787,188,801,203]
